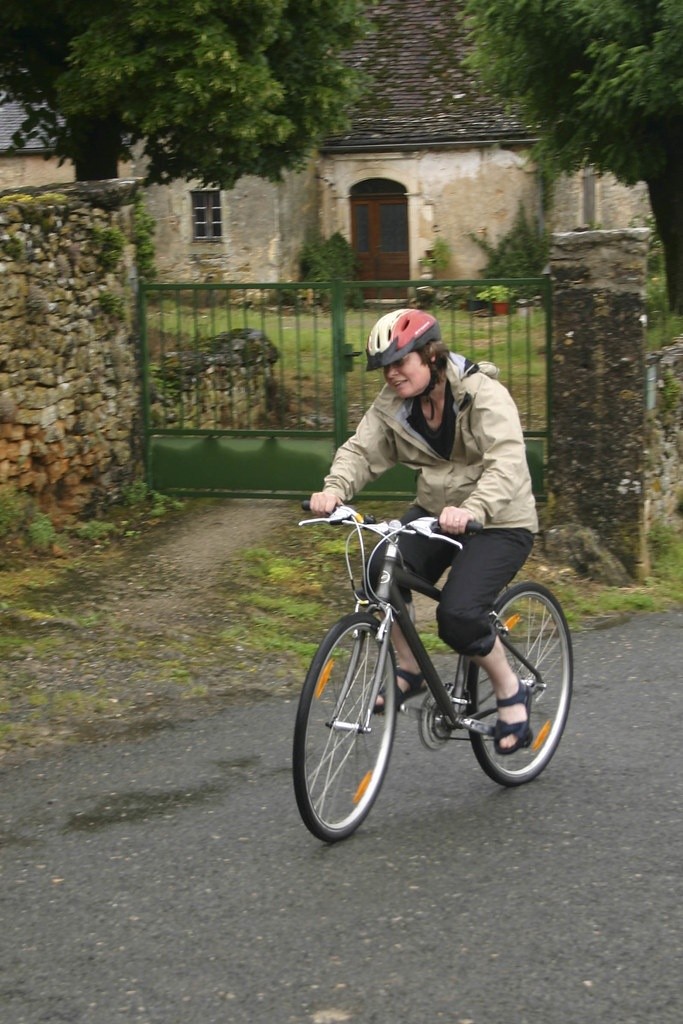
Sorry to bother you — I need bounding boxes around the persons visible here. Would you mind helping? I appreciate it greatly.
[308,308,540,754]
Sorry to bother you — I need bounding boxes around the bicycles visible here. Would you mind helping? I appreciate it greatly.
[292,500,575,843]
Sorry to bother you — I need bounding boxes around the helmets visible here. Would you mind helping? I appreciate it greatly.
[366,308,441,372]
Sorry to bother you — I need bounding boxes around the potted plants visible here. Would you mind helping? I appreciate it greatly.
[421,235,451,271]
[458,285,518,316]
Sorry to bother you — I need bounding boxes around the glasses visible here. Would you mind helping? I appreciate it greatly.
[380,355,411,371]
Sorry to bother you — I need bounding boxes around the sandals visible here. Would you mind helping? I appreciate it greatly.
[372,668,428,715]
[493,678,531,755]
